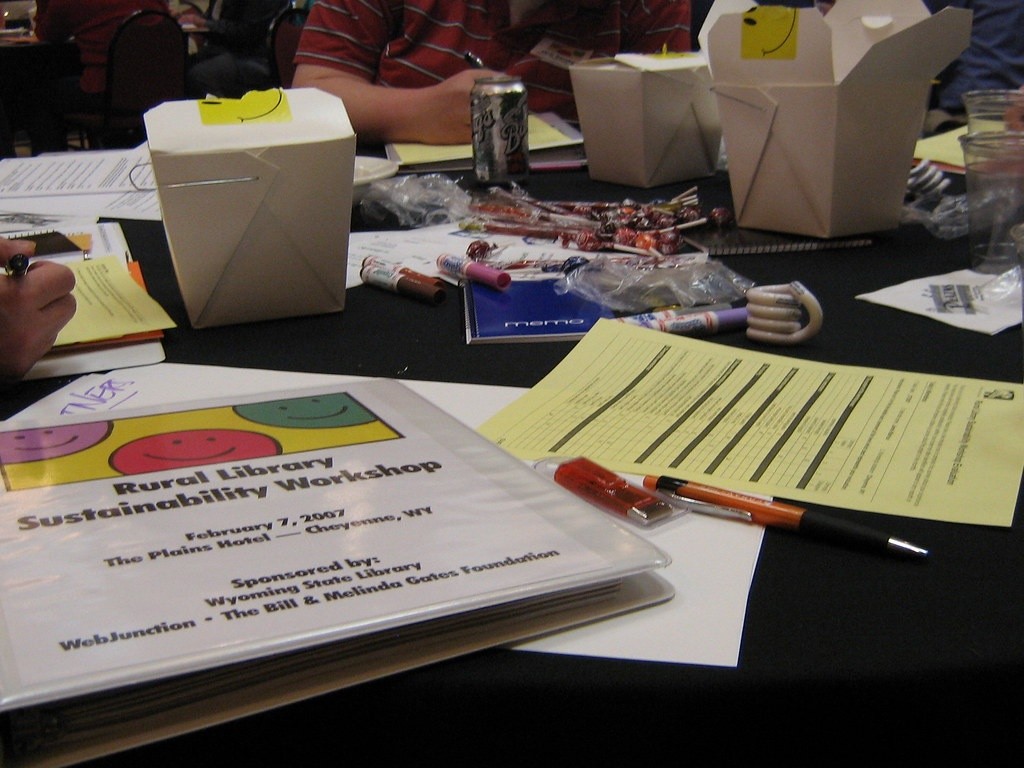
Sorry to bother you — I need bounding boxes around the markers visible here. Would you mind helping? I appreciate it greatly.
[359,255,448,305]
[437,254,512,292]
[613,299,757,335]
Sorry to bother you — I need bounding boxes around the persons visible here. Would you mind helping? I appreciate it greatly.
[291,0,692,144]
[193,0,284,98]
[0,235,76,381]
[692,0,1024,115]
[35,0,170,149]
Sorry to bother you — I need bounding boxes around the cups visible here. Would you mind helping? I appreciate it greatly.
[960,89,1024,129]
[957,129,1024,274]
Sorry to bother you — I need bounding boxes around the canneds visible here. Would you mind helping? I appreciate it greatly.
[470,76,530,185]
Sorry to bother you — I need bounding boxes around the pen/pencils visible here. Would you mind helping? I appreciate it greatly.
[462,49,484,68]
[642,472,935,567]
[5,253,30,275]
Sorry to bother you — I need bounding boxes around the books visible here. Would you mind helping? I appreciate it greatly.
[0,380,674,767]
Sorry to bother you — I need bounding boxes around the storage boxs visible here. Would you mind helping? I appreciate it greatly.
[144,88,355,329]
[572,0,980,241]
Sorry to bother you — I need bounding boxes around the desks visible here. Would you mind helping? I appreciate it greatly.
[0,158,1024,768]
